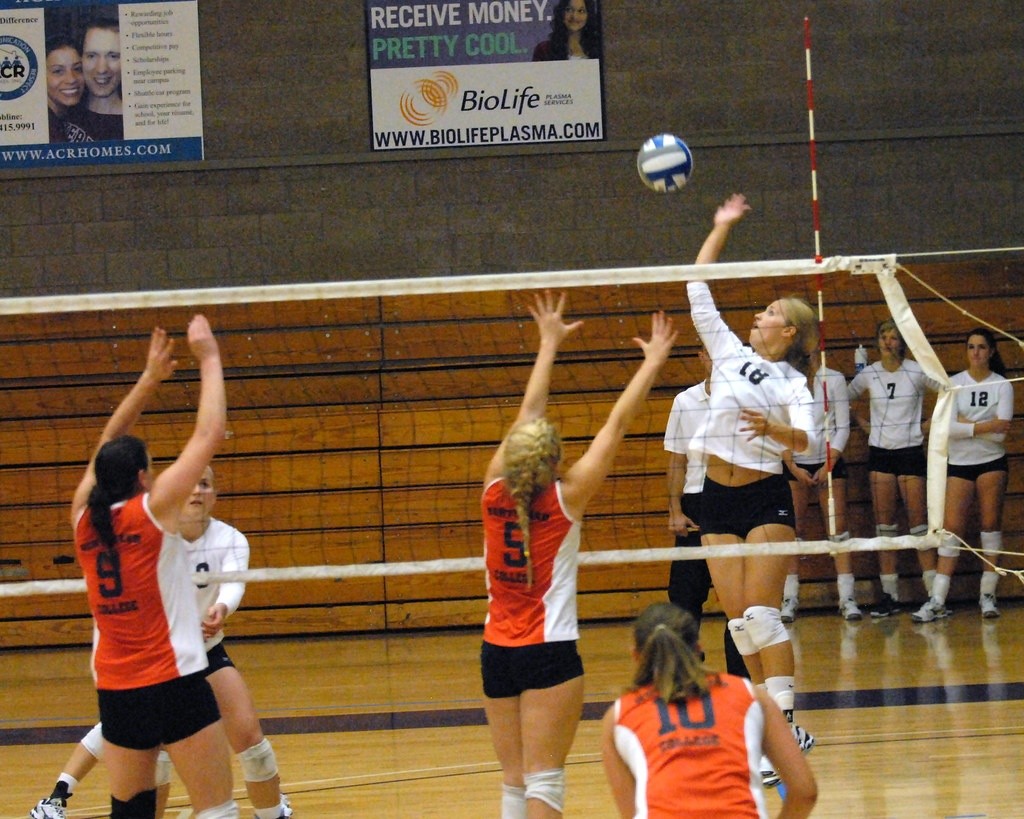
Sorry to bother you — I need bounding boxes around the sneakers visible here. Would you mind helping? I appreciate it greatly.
[872,617,900,637]
[760,722,815,788]
[982,619,998,641]
[870,598,901,617]
[840,621,862,637]
[912,602,948,621]
[979,593,1000,617]
[913,620,946,636]
[30,799,66,819]
[781,598,798,622]
[838,598,863,620]
[254,794,292,819]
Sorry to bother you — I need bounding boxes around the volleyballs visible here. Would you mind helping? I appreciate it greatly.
[636,132,695,193]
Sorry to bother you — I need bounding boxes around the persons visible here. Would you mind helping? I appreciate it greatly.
[844,319,952,617]
[686,194,818,789]
[912,326,1015,623]
[71,312,240,819]
[601,600,819,819]
[480,287,683,819]
[663,342,752,685]
[533,0,601,61]
[780,342,863,624]
[45,36,86,143]
[55,17,124,141]
[29,466,293,819]
